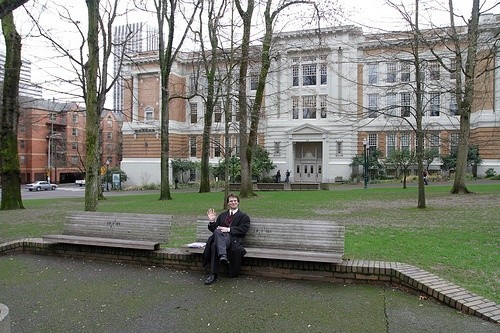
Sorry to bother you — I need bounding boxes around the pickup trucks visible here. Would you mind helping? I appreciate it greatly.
[75,178,86,186]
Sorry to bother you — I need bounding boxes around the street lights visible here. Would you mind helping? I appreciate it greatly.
[362,137,369,188]
[106,160,110,191]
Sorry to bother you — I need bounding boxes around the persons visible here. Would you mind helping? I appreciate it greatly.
[284,170,290,183]
[201,194,250,285]
[277,170,281,182]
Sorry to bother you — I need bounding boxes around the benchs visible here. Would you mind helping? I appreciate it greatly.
[42,210,173,250]
[290,184,319,190]
[180,218,345,264]
[229,184,241,190]
[257,183,284,191]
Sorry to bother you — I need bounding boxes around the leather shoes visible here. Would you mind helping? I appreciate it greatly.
[204,273,217,285]
[219,254,230,266]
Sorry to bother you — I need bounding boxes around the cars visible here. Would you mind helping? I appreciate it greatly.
[25,181,58,191]
